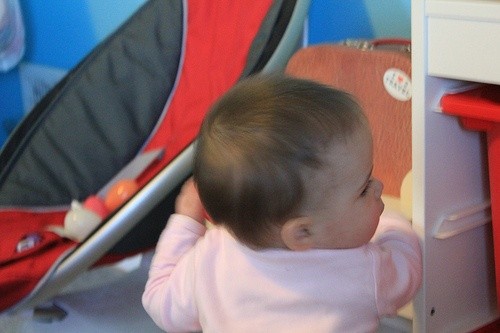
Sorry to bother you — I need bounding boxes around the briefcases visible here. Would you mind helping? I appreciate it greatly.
[282,37,412,200]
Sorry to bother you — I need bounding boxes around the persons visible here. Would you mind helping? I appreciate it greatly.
[142,73,423,333]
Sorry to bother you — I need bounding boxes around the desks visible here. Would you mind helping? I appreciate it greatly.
[410,0,500,333]
